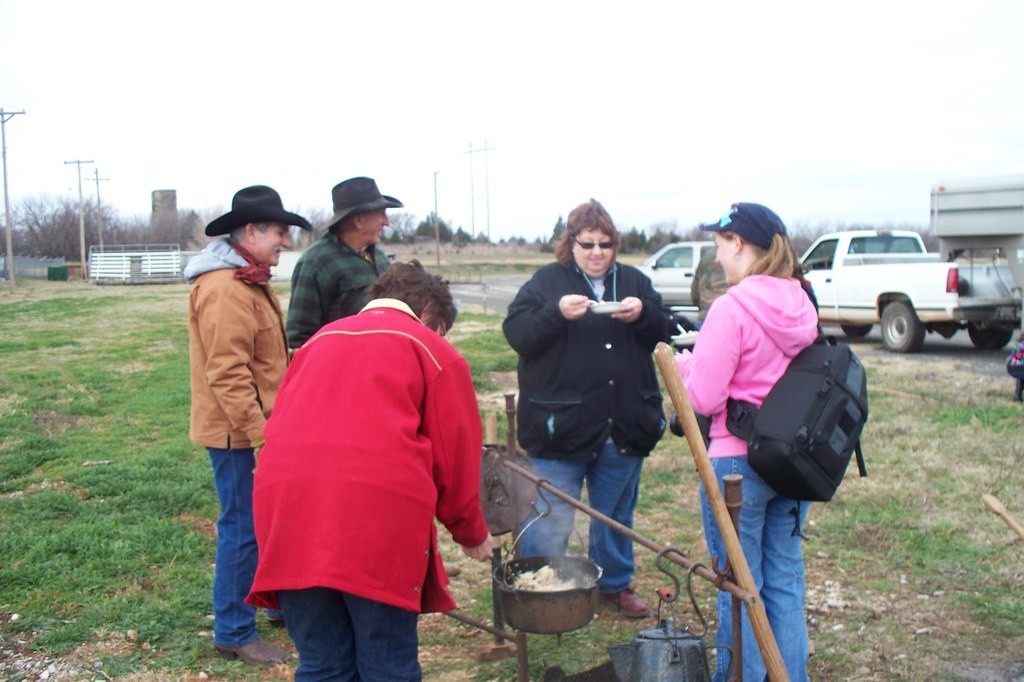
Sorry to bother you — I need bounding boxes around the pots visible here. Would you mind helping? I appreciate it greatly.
[492,512,603,634]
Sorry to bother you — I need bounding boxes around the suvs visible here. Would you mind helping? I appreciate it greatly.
[633,240,717,312]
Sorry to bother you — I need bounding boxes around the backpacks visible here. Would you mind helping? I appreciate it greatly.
[724,332,868,501]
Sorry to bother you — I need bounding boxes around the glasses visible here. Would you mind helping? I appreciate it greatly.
[571,235,613,248]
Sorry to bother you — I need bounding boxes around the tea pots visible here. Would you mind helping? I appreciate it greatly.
[608,590,734,682]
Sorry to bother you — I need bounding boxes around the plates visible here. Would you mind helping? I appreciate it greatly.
[588,301,622,314]
[671,334,699,346]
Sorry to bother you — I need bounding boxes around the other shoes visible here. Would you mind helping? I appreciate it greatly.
[443,561,462,576]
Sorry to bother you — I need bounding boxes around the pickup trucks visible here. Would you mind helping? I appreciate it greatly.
[798,230,1021,353]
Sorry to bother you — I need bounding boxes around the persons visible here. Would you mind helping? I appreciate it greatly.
[243,260,493,682]
[285,176,404,349]
[674,202,820,682]
[500,198,671,618]
[183,185,309,665]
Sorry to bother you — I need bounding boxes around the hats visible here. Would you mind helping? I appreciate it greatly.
[317,177,403,232]
[205,186,311,237]
[700,202,786,250]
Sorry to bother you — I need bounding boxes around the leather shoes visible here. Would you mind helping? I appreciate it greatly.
[265,614,284,629]
[215,637,290,667]
[600,586,650,617]
